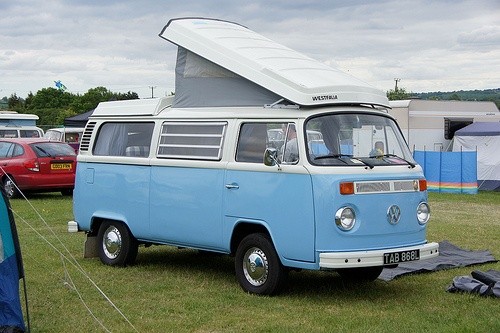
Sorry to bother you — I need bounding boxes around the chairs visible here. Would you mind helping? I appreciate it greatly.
[125,145,149,157]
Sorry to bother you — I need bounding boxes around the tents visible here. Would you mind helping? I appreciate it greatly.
[444,121,500,194]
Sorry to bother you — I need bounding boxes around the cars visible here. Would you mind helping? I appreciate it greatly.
[42,126,85,156]
[0,137,77,199]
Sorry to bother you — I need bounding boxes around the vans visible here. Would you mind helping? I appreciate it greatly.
[65,15,440,296]
[0,110,46,137]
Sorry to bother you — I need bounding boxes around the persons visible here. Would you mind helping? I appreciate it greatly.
[369,141,385,157]
[280,123,314,164]
